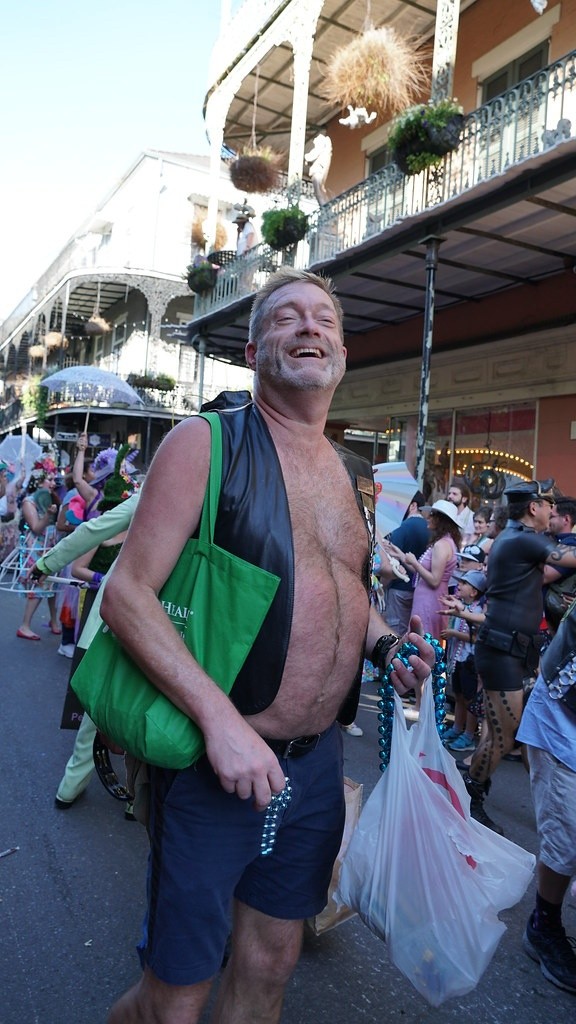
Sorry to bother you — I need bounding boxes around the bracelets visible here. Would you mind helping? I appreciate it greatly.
[372,634,403,668]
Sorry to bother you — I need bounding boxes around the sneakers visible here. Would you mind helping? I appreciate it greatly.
[442,728,465,742]
[448,734,476,751]
[523,913,576,993]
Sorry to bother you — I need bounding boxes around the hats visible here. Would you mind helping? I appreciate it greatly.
[419,499,487,592]
[89,444,137,511]
[504,478,556,503]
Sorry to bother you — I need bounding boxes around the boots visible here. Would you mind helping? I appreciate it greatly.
[463,772,504,836]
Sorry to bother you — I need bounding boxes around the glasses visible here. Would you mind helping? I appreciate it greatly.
[429,512,436,516]
[488,520,494,522]
[541,503,555,507]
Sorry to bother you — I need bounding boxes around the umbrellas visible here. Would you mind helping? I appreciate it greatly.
[41,364,145,448]
[371,461,419,544]
[0,435,43,469]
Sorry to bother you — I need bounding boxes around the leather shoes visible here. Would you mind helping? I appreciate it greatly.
[502,754,523,761]
[456,760,470,771]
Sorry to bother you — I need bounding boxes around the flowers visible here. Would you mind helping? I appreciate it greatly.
[181,261,212,279]
[387,97,464,148]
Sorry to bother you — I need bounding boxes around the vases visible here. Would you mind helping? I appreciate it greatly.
[188,270,217,294]
[393,114,462,176]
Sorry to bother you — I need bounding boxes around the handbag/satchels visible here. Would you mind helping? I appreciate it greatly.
[69,412,283,768]
[308,673,535,1008]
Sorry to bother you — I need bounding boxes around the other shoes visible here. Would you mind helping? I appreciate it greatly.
[402,707,420,721]
[47,620,61,634]
[125,804,136,820]
[58,644,74,657]
[54,797,70,808]
[17,629,40,640]
[344,724,363,736]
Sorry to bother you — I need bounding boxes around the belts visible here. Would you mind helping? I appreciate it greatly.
[262,734,324,760]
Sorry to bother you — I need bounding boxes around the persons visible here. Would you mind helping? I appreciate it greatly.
[99,266,434,1024]
[305,134,332,209]
[514,599,576,990]
[0,434,142,821]
[232,214,261,296]
[339,480,576,837]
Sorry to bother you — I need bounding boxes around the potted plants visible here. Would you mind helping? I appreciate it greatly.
[261,204,308,248]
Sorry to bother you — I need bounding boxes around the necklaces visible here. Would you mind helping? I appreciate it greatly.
[378,633,448,773]
[447,602,481,674]
[411,544,432,586]
[261,778,292,854]
